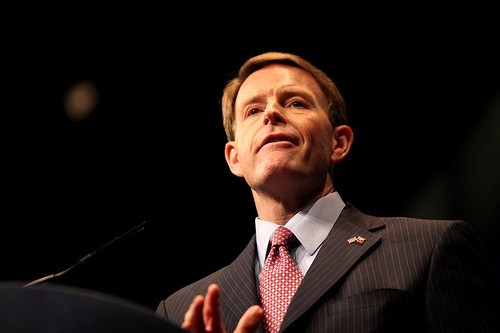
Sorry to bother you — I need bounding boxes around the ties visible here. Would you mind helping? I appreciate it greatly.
[258,225,303,333]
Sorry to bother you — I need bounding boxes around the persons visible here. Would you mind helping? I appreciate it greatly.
[154,50,500,333]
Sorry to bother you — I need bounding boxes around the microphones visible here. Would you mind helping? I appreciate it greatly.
[22,219,147,287]
